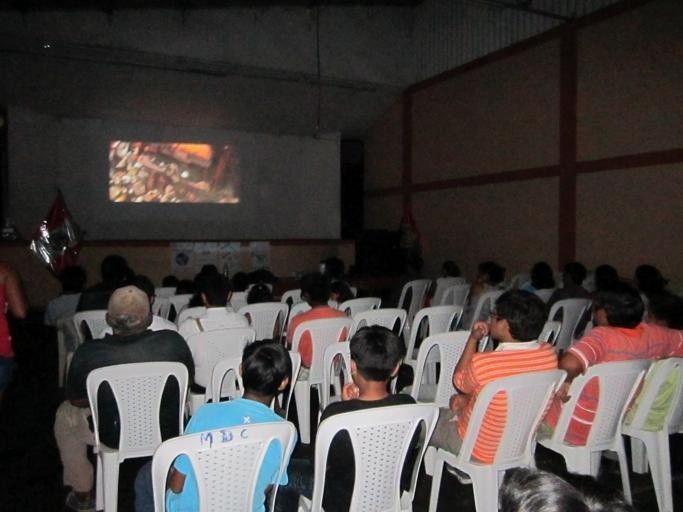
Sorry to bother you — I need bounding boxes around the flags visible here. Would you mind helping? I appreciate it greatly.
[29,192,85,279]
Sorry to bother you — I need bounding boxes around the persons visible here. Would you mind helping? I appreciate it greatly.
[1,255,682,511]
[108,140,242,204]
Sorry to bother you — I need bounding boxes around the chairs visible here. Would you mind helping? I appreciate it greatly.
[26,266,683,512]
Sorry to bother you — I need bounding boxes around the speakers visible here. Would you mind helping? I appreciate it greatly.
[341,140,364,239]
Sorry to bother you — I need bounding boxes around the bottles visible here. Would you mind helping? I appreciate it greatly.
[223,263,228,281]
[345,383,359,399]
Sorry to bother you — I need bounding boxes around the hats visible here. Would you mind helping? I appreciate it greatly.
[108,285,149,330]
